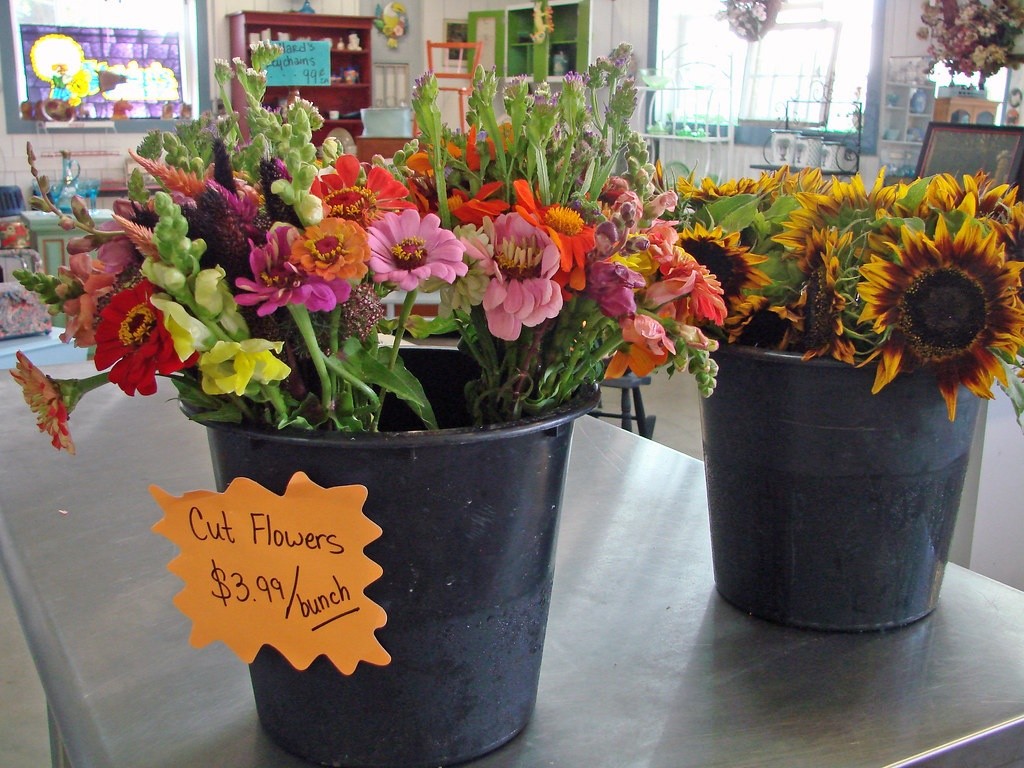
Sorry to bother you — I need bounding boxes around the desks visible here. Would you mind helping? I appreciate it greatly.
[1,365,1024,768]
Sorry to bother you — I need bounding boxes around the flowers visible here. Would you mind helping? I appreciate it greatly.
[714,0,785,42]
[0,41,724,435]
[651,158,1024,420]
[916,1,1023,78]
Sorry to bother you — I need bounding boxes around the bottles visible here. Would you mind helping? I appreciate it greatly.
[911,87,927,114]
[336,31,363,51]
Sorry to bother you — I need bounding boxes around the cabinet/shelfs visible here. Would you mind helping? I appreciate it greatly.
[634,44,732,188]
[936,98,1002,126]
[225,10,376,149]
[468,0,593,86]
[877,82,938,163]
[22,209,134,327]
[749,87,862,181]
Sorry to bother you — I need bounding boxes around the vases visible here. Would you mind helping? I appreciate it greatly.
[694,331,985,631]
[176,391,601,766]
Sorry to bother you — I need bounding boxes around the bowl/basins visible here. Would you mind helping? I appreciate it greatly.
[360,108,412,137]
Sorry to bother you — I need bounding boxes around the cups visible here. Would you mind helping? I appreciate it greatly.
[820,141,846,170]
[770,128,795,166]
[32,177,101,217]
[329,111,339,120]
[796,137,824,167]
[249,28,291,45]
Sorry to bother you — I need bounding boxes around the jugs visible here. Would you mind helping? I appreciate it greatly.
[52,150,81,214]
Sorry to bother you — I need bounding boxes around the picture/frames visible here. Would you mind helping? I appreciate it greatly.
[444,19,471,67]
[912,122,1024,207]
